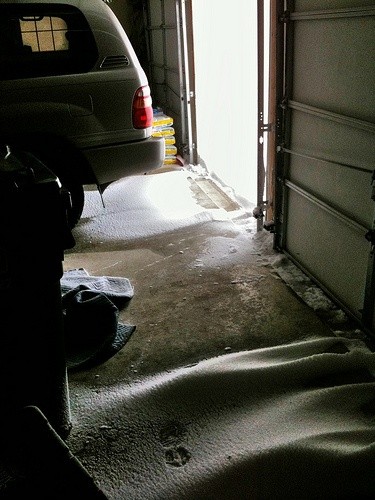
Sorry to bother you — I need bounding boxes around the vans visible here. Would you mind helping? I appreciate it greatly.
[0,0,163,235]
